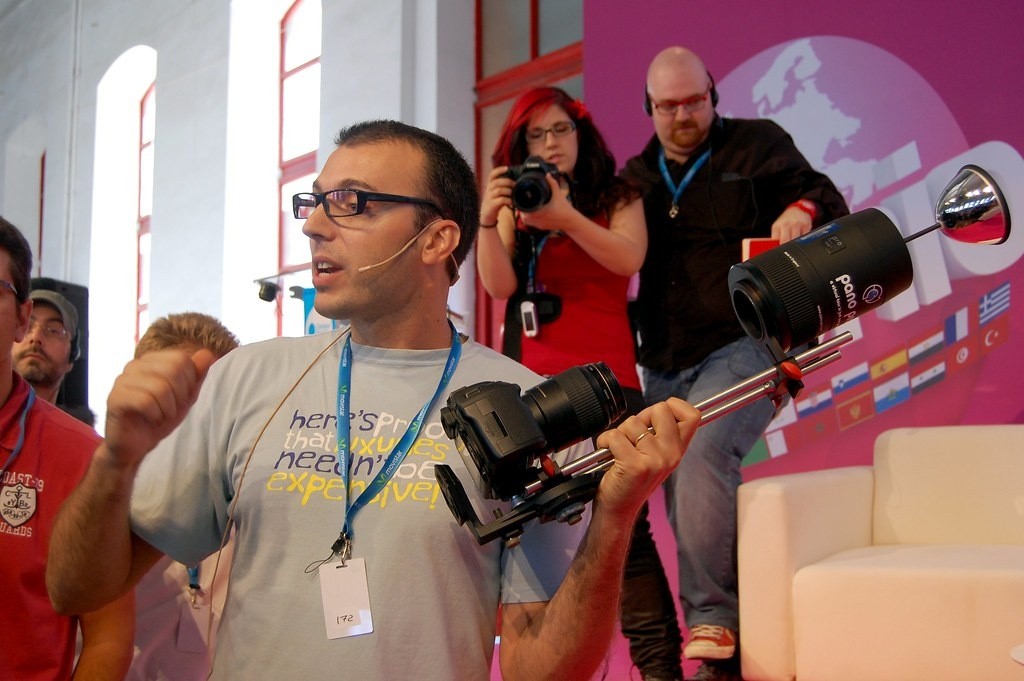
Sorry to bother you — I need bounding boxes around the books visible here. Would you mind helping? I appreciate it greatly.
[742,238,781,264]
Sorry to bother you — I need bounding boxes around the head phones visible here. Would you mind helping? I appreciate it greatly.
[643,69,718,117]
[70,327,80,362]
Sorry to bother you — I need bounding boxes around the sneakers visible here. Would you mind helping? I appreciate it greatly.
[686,624,736,659]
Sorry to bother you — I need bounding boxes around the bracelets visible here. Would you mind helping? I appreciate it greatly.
[479,220,498,229]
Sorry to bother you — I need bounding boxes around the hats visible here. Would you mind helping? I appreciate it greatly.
[28,289,80,336]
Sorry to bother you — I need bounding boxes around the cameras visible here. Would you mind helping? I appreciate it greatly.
[504,154,560,212]
[439,361,628,502]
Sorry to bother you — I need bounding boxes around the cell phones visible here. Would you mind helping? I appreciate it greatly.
[521,301,539,337]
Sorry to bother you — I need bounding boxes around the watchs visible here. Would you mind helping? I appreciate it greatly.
[788,197,817,221]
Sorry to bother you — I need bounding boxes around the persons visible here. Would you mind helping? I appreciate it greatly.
[72,311,245,681]
[45,118,705,681]
[0,216,136,681]
[476,86,686,681]
[11,288,80,418]
[622,45,852,681]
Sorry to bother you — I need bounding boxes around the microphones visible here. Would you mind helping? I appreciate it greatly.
[359,221,435,273]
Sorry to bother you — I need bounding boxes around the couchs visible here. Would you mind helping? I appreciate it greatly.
[736,423,1024,681]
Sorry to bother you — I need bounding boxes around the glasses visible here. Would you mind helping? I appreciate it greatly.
[0,280,18,296]
[648,85,710,116]
[292,188,448,220]
[525,120,573,144]
[28,321,66,340]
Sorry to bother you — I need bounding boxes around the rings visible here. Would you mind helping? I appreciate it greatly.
[634,432,651,447]
